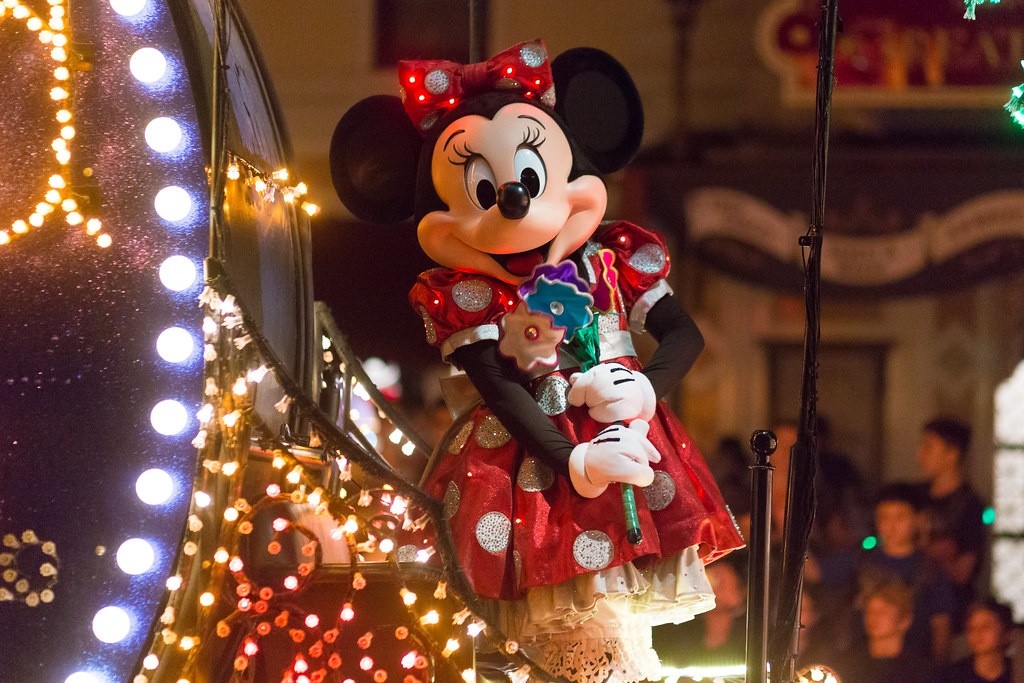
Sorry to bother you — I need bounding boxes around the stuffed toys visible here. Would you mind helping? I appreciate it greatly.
[328,40,746,683]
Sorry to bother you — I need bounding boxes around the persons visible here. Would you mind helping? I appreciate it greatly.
[698,415,1024,683]
